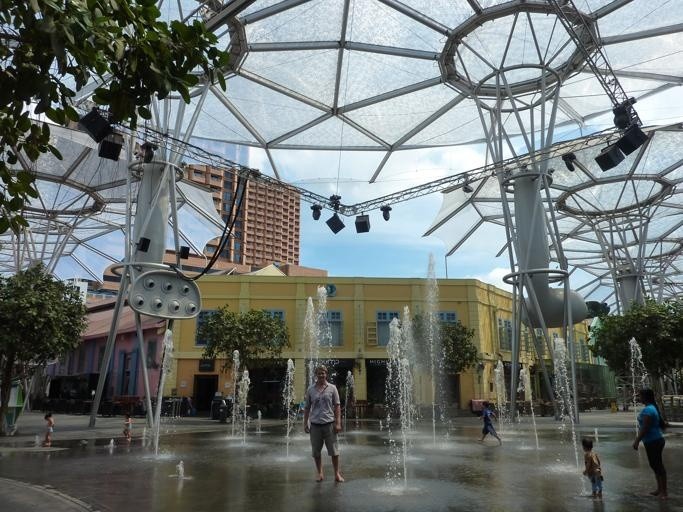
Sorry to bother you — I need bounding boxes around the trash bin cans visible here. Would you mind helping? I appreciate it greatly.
[212,399,234,423]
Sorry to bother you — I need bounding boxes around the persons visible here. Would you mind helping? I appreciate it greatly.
[43,413,55,446]
[123,414,133,441]
[303,365,345,483]
[478,401,502,446]
[582,438,603,497]
[632,389,668,499]
